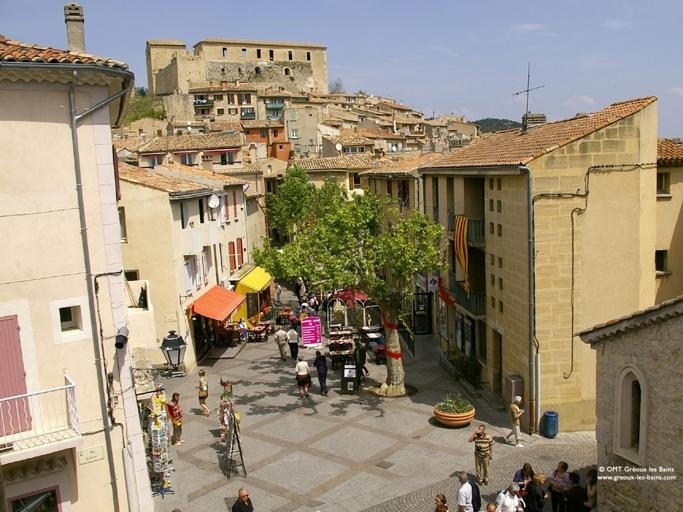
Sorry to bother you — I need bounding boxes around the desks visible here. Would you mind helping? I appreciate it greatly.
[220,300,293,347]
[326,323,385,369]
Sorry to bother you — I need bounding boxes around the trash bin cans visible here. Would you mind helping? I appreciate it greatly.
[545,412,558,439]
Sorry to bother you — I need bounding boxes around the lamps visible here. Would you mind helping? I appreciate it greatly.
[128,328,189,389]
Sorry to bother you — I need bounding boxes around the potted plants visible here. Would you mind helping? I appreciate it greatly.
[432,390,477,428]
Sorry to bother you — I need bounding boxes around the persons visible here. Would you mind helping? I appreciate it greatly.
[502,393,527,448]
[430,460,598,512]
[467,423,493,487]
[237,271,334,400]
[167,389,187,448]
[229,483,254,512]
[193,368,210,418]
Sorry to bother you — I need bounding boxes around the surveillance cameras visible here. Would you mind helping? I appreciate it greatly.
[115,327,129,349]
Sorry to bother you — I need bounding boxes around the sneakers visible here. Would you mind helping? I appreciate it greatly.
[301,393,329,399]
[201,410,211,417]
[504,436,524,448]
[479,481,488,486]
[174,440,184,446]
